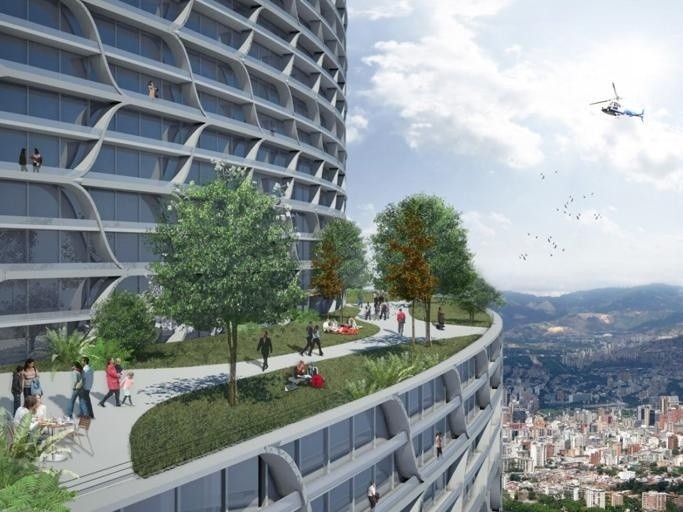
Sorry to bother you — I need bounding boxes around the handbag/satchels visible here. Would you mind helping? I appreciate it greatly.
[31,379,42,396]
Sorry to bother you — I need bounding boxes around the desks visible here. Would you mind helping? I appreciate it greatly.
[37,419,74,462]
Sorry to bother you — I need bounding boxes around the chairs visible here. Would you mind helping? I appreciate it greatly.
[5,421,16,448]
[71,415,93,453]
